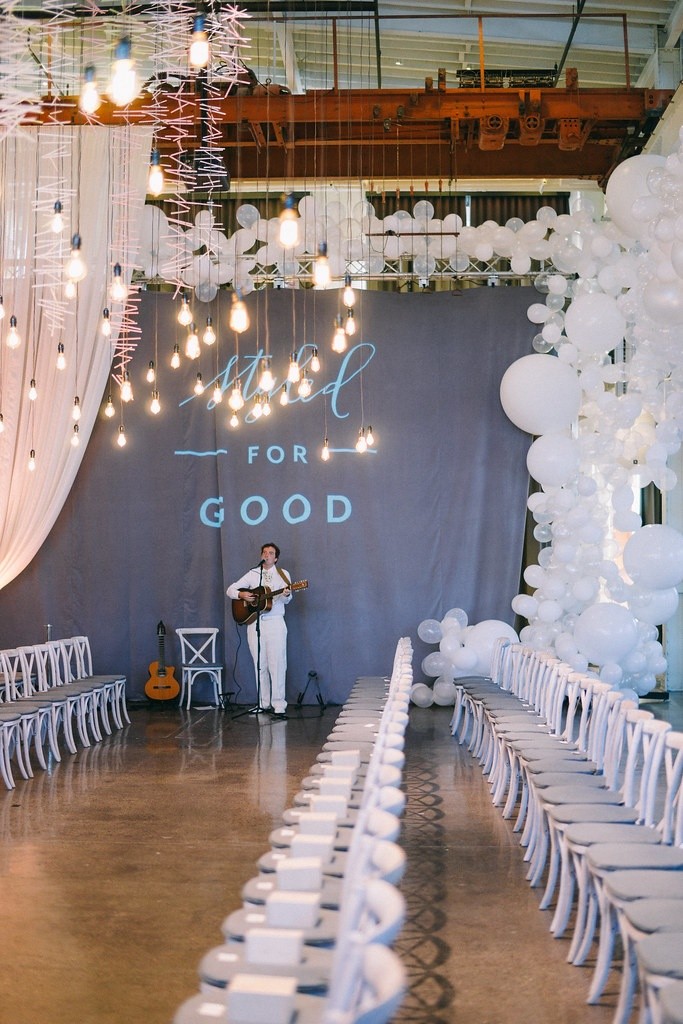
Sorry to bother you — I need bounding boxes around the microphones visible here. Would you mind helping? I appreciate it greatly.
[258,559,267,568]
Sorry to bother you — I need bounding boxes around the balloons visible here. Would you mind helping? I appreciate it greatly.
[132,196,516,302]
[499,125,683,709]
[410,608,520,708]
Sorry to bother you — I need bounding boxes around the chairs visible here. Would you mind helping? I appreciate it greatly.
[172,635,414,1024]
[0,636,131,790]
[175,627,225,711]
[448,636,683,1024]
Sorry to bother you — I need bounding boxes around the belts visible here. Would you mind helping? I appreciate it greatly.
[261,615,283,620]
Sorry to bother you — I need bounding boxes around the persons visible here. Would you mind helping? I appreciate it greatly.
[226,542,293,714]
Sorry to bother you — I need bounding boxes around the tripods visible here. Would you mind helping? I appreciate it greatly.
[231,563,291,720]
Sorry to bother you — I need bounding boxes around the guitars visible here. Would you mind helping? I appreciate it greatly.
[145,620,180,703]
[231,578,308,625]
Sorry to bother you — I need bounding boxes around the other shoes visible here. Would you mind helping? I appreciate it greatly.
[248,705,272,713]
[275,712,288,720]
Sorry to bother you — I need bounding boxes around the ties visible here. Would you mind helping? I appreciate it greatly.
[265,572,271,588]
